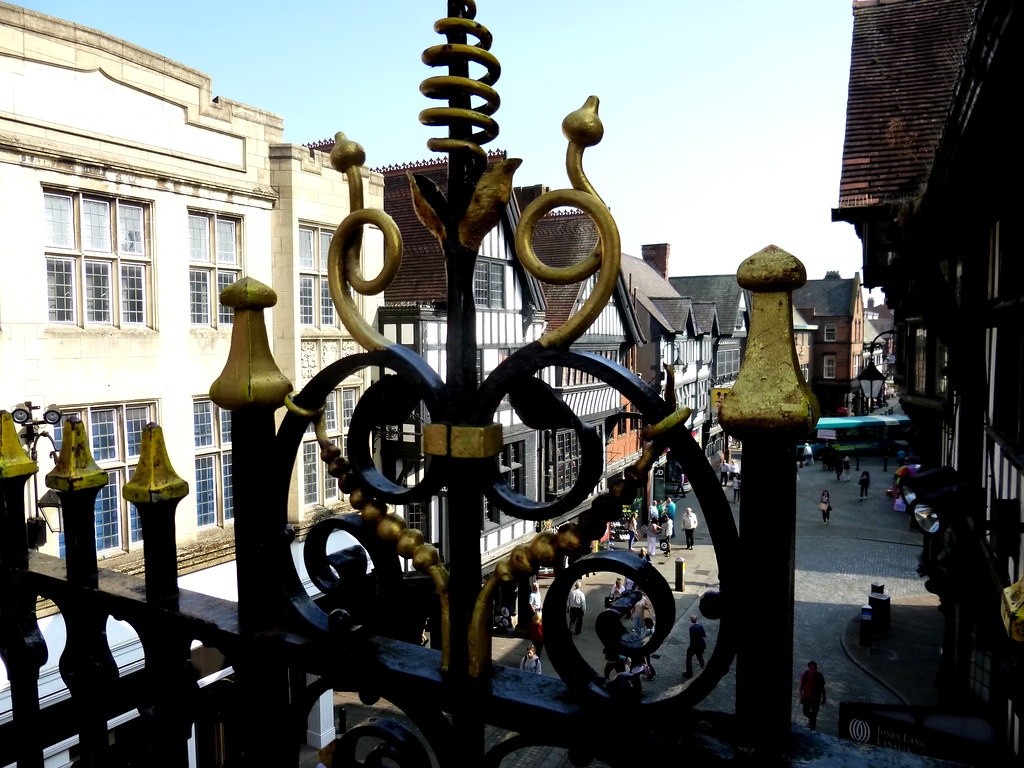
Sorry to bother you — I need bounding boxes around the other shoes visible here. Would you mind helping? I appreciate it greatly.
[629,547,635,551]
[574,630,580,635]
[822,522,826,525]
[859,497,862,500]
[827,519,830,523]
[689,546,694,549]
[686,545,690,548]
[663,550,671,557]
[642,676,654,681]
[681,668,692,677]
[865,496,867,500]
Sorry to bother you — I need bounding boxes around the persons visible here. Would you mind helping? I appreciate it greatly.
[475,372,933,742]
